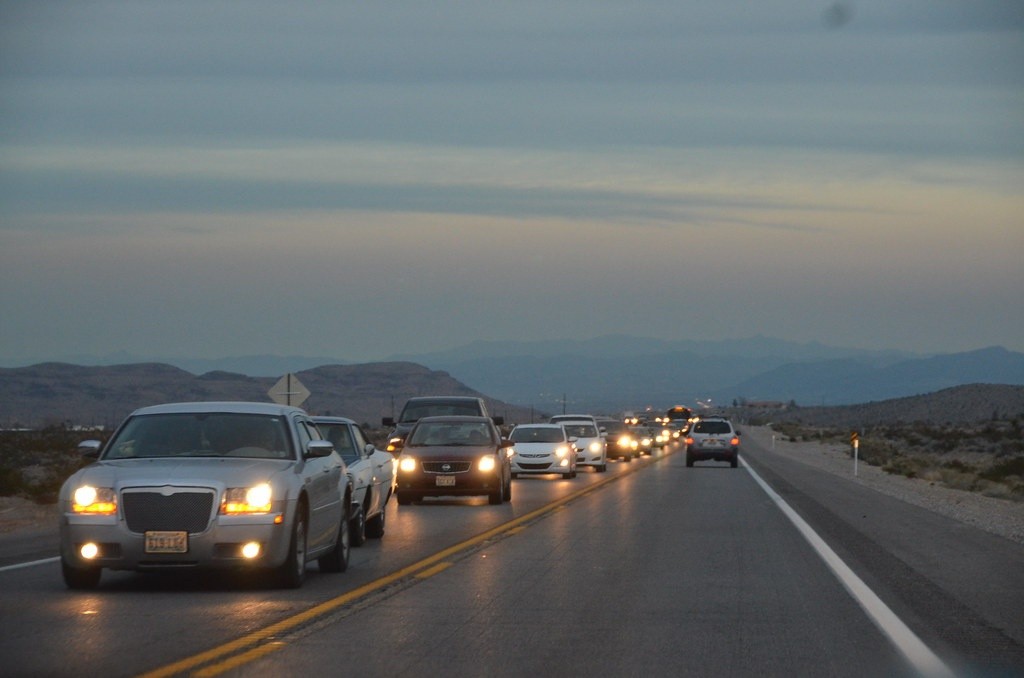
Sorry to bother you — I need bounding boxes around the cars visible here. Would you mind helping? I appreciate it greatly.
[596,404,701,463]
[549,420,608,475]
[501,423,579,480]
[389,417,517,505]
[305,413,398,546]
[56,401,357,593]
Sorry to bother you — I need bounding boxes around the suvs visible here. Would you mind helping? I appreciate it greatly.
[381,396,504,495]
[685,418,741,468]
[548,414,598,424]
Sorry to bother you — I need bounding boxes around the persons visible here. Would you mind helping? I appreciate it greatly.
[469,430,481,442]
[257,427,285,457]
[327,427,347,446]
